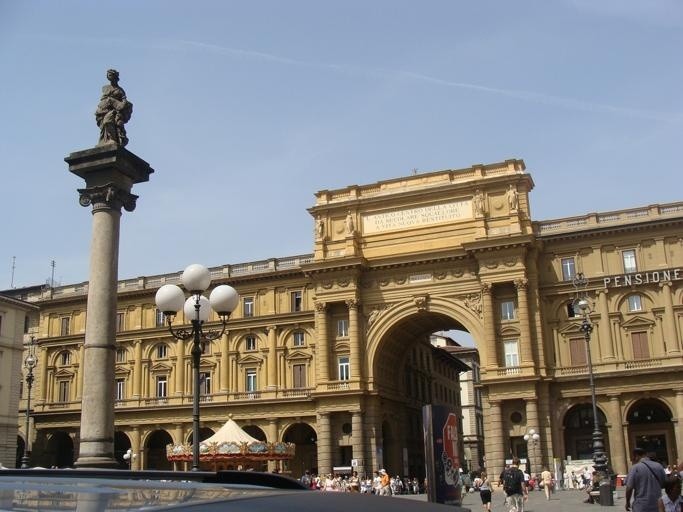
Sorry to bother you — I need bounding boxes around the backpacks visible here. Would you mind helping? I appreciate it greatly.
[502,471,516,492]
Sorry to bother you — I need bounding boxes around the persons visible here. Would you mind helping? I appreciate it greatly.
[539,465,551,500]
[581,466,590,488]
[506,184,519,210]
[502,456,525,512]
[471,189,486,219]
[523,470,530,494]
[315,215,324,239]
[93,68,128,147]
[345,210,353,236]
[298,467,468,502]
[562,470,569,490]
[656,475,683,511]
[570,471,577,489]
[624,447,668,511]
[477,471,493,512]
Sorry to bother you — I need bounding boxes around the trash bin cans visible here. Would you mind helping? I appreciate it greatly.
[599,480,612,506]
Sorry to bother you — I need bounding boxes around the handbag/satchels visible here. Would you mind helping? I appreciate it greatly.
[538,480,544,488]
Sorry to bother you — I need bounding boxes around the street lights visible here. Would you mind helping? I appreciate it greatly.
[21,355,38,469]
[524,430,540,491]
[155,265,239,471]
[578,300,608,477]
[124,450,137,470]
